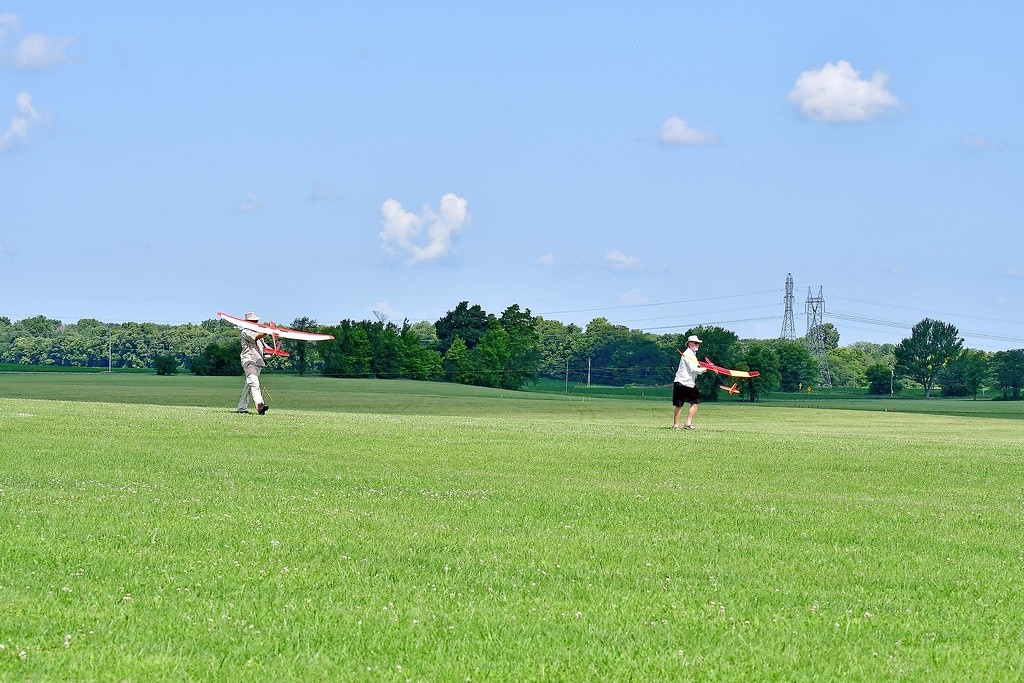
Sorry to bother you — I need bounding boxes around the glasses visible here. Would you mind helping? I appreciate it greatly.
[694,342,699,345]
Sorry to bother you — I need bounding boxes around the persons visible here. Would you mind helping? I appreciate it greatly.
[672,335,711,430]
[235,313,270,415]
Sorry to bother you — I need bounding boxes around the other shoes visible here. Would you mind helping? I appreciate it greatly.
[237,411,248,413]
[684,422,694,429]
[673,424,679,430]
[258,402,268,415]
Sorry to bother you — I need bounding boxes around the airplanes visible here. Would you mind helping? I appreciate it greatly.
[676,348,760,395]
[217,311,334,357]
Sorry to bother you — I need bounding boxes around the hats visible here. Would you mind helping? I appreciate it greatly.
[245,311,261,320]
[685,335,703,347]
